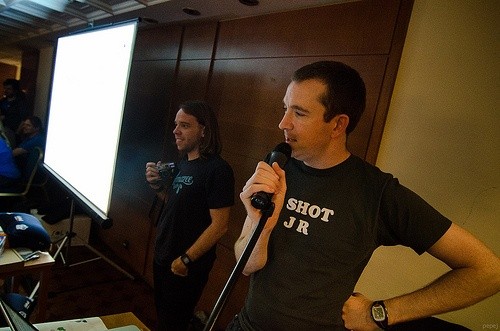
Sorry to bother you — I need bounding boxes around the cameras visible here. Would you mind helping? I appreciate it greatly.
[151,162,178,181]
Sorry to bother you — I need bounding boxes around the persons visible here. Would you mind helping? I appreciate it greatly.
[146,101,236,331]
[13,117,46,185]
[0,130,20,194]
[0,78,29,145]
[233,60,500,331]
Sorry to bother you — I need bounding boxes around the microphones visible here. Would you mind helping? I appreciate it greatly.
[250,142,292,209]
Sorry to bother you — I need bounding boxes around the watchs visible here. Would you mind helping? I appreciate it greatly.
[371,300,388,330]
[180,253,192,267]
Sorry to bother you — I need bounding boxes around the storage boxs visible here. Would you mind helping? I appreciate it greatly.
[29,208,92,247]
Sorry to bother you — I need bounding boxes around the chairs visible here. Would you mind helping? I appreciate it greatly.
[0,146,43,210]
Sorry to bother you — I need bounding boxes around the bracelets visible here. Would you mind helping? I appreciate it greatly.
[148,184,164,193]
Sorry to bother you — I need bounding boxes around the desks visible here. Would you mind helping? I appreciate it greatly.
[0,245,56,322]
[0,312,151,331]
